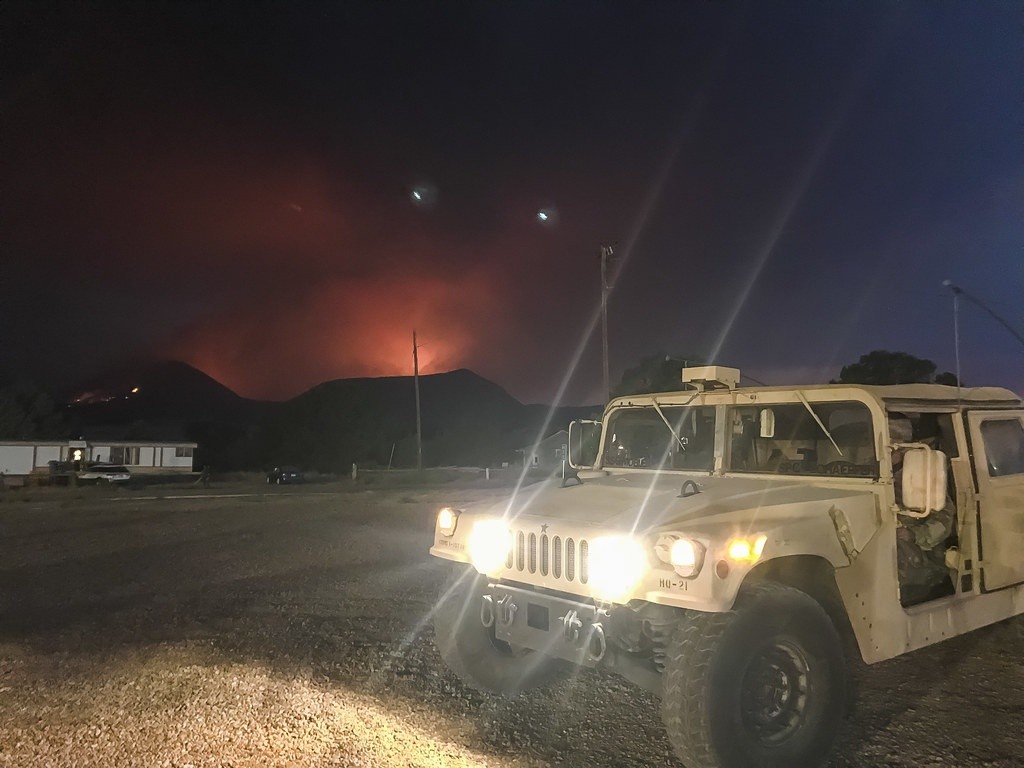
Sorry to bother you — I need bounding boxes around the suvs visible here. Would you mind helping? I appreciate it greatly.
[433,364,1024,768]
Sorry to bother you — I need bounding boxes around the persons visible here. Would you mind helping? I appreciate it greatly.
[886,411,956,606]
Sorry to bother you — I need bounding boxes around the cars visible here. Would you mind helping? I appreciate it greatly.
[266,465,303,486]
[76,464,133,487]
